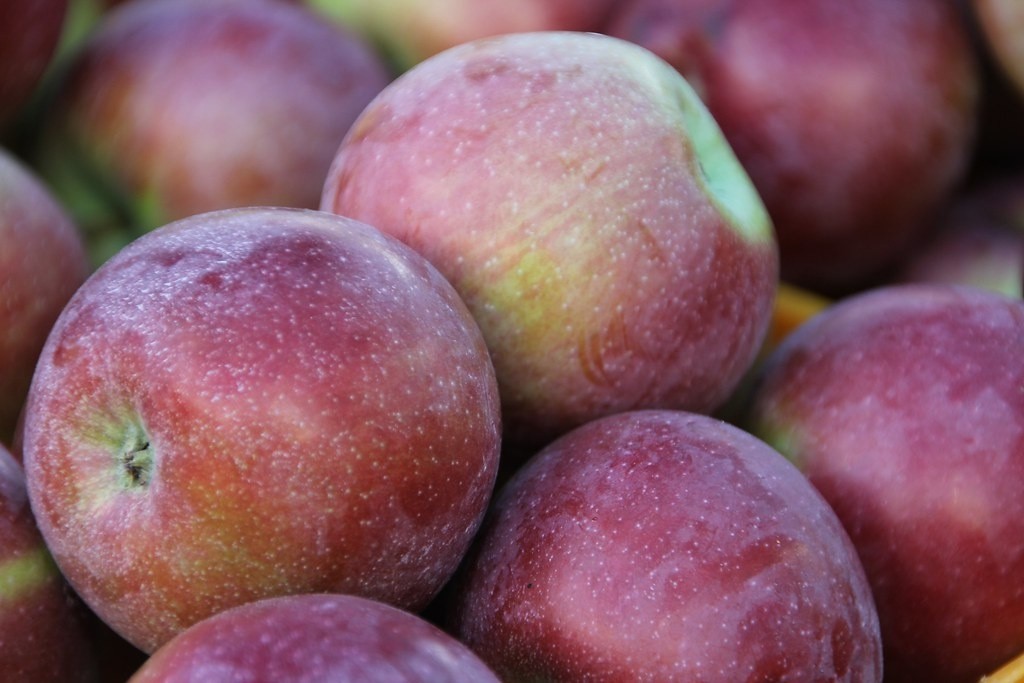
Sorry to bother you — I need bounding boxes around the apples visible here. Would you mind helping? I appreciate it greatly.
[0,0,1024,682]
